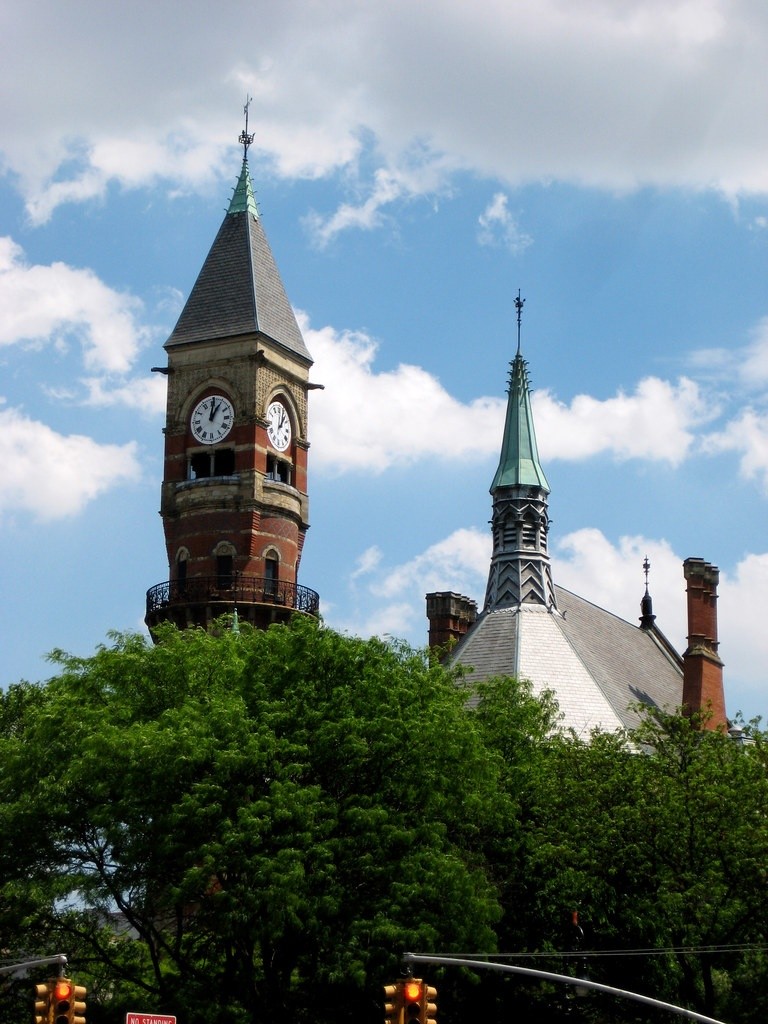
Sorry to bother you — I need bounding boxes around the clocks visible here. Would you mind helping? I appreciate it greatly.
[190,394,235,445]
[266,402,292,452]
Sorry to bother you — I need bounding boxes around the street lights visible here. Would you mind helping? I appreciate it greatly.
[554,907,600,1023]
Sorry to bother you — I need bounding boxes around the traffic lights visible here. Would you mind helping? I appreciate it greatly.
[33,977,87,1024]
[384,978,436,1024]
